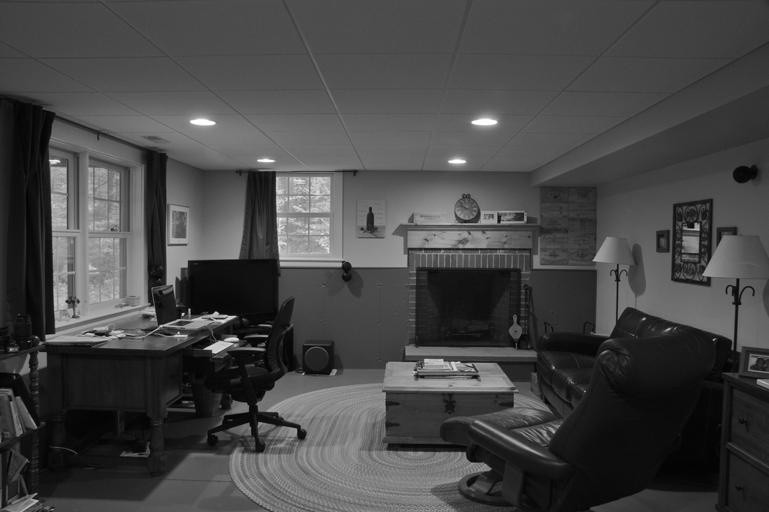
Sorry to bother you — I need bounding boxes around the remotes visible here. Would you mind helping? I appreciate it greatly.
[201,312,229,321]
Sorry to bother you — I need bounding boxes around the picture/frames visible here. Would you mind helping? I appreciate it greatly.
[716,227,738,248]
[353,199,387,239]
[738,345,769,379]
[670,198,715,289]
[166,204,191,247]
[655,230,669,253]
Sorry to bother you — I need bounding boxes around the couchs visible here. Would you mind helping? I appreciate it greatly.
[534,307,732,470]
[440,330,717,512]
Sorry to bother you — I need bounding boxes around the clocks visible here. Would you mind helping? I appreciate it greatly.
[453,193,479,224]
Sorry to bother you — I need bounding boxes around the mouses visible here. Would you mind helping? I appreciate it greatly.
[224,337,239,343]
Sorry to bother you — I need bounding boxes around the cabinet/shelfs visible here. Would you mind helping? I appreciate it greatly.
[720,370,769,512]
[0,342,51,512]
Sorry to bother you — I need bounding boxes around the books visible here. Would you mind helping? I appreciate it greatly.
[413,359,480,379]
[0,442,28,483]
[0,492,57,512]
[0,389,37,443]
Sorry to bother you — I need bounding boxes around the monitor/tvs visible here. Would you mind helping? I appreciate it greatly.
[188,259,279,317]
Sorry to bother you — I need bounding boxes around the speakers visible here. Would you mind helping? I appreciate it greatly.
[303,340,335,374]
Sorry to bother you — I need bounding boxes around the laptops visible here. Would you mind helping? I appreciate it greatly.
[151,284,210,330]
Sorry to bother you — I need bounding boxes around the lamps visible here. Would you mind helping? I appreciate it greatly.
[593,235,636,325]
[702,234,769,372]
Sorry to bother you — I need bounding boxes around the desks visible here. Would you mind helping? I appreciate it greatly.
[38,315,240,453]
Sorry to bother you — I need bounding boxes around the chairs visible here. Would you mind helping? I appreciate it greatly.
[203,297,308,454]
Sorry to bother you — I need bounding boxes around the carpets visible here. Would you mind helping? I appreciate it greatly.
[228,382,567,512]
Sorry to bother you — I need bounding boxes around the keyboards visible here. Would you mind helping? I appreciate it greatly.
[203,340,233,355]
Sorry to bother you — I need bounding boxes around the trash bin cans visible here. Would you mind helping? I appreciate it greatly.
[193,388,222,417]
